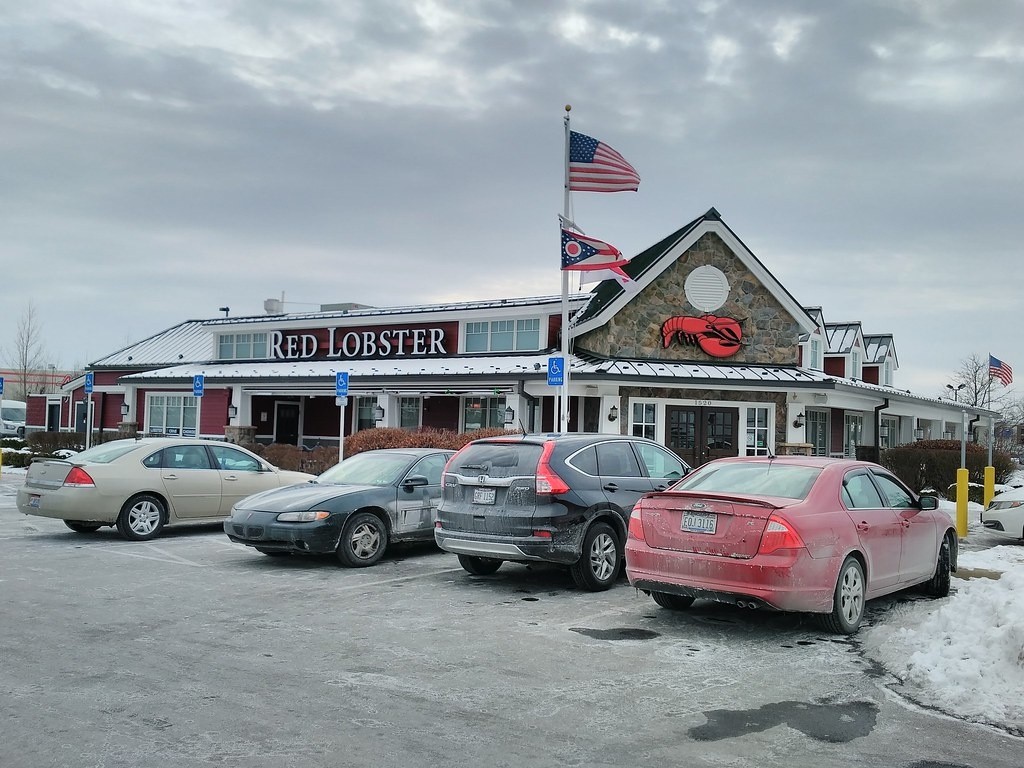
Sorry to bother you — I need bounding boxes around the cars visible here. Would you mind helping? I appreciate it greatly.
[625,455,960,633]
[223,449,459,567]
[980,486,1024,540]
[16,439,317,541]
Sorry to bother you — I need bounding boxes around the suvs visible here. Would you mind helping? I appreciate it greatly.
[433,434,697,591]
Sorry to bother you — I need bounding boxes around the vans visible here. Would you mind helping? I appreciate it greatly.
[0,399,26,437]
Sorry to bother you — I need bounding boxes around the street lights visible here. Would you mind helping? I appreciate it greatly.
[946,383,966,401]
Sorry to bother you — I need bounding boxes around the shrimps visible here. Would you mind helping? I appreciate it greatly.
[661,314,749,358]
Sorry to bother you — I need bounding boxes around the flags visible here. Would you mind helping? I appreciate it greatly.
[989,355,1012,388]
[561,229,631,270]
[579,266,640,295]
[568,130,641,192]
